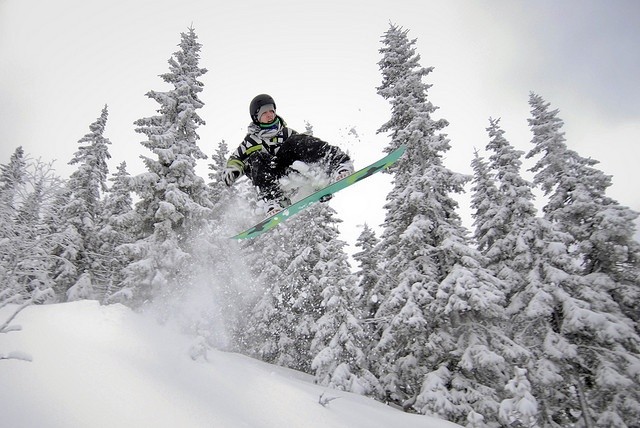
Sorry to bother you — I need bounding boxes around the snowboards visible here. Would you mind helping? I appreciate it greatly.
[228,146,404,239]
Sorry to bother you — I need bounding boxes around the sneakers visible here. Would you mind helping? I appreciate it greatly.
[332,158,354,180]
[263,199,290,217]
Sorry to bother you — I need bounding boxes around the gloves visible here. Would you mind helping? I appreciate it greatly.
[222,166,241,187]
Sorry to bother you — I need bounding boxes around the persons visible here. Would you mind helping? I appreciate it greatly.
[224,94,354,214]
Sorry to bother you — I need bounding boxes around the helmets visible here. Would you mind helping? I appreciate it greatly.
[250,94,277,124]
